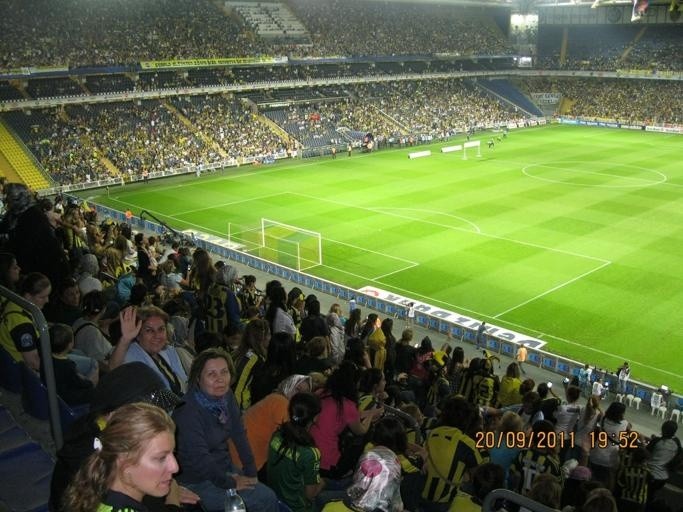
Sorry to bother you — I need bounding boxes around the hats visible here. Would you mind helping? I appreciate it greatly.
[85,361,186,422]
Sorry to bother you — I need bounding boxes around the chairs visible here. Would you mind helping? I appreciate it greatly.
[59,393,78,442]
[22,368,49,421]
[0,344,25,393]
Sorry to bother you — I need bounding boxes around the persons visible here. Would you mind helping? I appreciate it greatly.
[0,0,683,186]
[0,177,682,512]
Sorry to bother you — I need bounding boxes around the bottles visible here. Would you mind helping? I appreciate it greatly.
[222,488,246,512]
